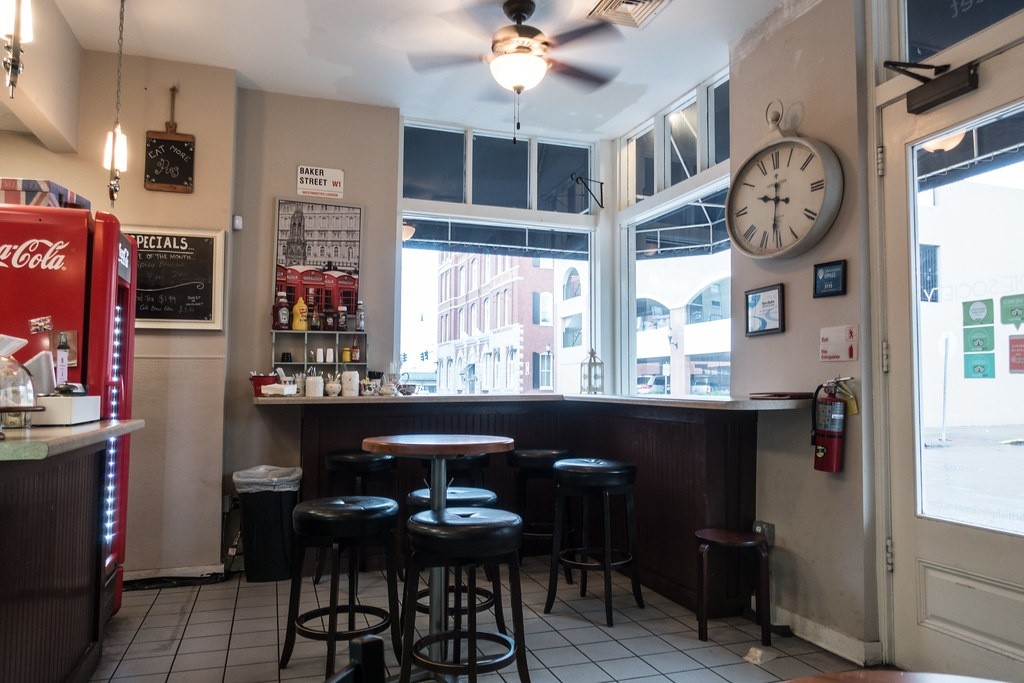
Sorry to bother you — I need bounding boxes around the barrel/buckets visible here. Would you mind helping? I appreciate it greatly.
[249,376,276,397]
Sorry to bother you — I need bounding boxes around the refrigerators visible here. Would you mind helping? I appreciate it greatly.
[0,204,139,623]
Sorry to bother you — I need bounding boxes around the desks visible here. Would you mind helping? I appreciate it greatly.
[363,434,516,683]
[256,392,808,618]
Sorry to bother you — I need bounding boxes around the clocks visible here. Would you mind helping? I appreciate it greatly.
[725,100,843,262]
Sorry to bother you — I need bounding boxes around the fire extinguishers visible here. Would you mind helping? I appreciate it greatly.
[811,373,854,473]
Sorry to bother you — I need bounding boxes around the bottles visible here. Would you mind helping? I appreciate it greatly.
[324,304,334,330]
[292,297,308,330]
[58,332,70,349]
[272,292,289,330]
[356,301,365,332]
[342,348,351,362]
[311,302,321,330]
[351,335,360,362]
[337,306,348,331]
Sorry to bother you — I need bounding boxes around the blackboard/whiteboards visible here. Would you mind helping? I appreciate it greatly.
[144,122,196,193]
[120,224,225,330]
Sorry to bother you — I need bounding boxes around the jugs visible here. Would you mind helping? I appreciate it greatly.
[378,381,397,396]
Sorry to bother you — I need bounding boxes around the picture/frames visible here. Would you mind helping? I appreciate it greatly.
[813,260,847,298]
[745,281,785,335]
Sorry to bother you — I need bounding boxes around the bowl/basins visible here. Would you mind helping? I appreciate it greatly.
[368,371,384,379]
[400,385,416,395]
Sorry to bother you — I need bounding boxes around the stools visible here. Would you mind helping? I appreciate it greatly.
[401,453,493,582]
[544,457,646,628]
[695,529,772,647]
[315,451,404,596]
[280,496,405,683]
[400,507,533,683]
[506,449,576,585]
[399,487,507,660]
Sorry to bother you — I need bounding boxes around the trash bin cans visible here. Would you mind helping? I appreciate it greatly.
[233,465,303,583]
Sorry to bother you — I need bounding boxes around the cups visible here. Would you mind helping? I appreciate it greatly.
[325,383,341,396]
[341,371,359,396]
[361,383,374,395]
[305,376,324,396]
[295,379,305,397]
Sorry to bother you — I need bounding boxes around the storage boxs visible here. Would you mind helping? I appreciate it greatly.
[0,178,90,206]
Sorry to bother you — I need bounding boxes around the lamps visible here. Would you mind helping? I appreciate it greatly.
[486,1,550,143]
[0,0,35,99]
[103,0,128,201]
